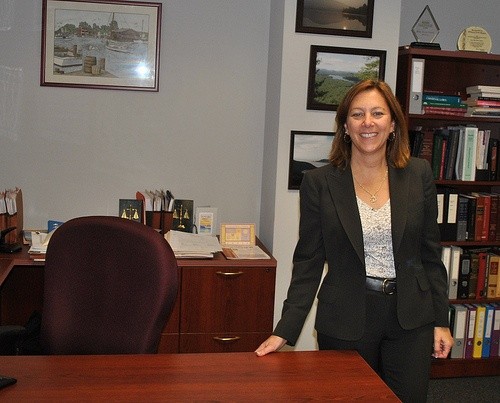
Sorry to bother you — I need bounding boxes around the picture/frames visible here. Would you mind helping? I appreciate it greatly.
[40,0,162,91]
[295,0,374,38]
[288,130,336,190]
[306,45,387,111]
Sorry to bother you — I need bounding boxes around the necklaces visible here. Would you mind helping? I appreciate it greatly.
[354,173,387,202]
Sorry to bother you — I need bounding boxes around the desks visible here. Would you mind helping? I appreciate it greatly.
[0,349,401,403]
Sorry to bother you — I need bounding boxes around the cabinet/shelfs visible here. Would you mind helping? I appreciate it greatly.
[395,48,500,378]
[0,234,277,352]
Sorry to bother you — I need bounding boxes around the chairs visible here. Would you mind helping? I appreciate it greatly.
[0,216,178,355]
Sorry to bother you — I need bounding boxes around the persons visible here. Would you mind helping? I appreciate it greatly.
[222,246,270,259]
[255,78,455,403]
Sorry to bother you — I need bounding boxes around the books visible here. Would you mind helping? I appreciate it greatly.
[24,230,48,246]
[451,127,500,181]
[422,90,468,117]
[141,189,174,211]
[408,126,459,180]
[436,191,500,241]
[27,231,49,261]
[440,246,500,299]
[465,86,500,118]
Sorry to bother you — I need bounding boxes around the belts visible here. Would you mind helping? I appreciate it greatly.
[365,277,397,294]
